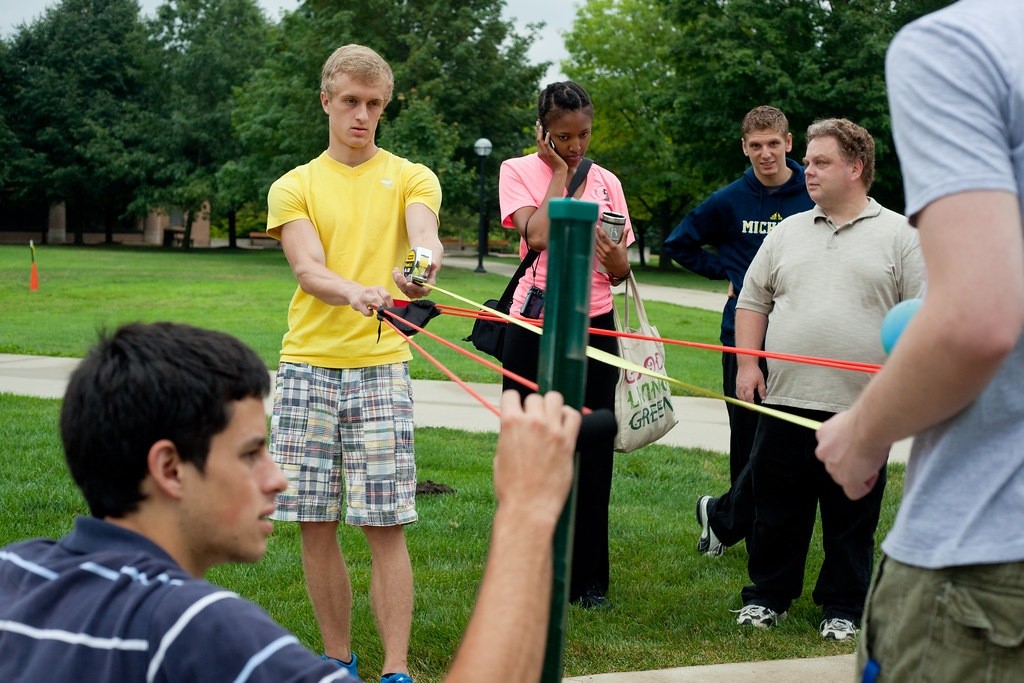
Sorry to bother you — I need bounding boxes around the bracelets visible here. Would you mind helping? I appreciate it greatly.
[609,272,630,280]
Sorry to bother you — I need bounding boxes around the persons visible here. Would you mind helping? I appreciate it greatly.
[733,119,924,641]
[664,105,816,563]
[814,1,1024,683]
[266,43,445,683]
[499,80,637,611]
[0,321,582,683]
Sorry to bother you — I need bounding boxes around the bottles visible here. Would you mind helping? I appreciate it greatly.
[594,212,627,275]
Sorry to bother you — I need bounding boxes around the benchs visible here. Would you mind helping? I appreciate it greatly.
[249,232,279,248]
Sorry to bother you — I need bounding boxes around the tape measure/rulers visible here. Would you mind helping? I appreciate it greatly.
[402,246,823,433]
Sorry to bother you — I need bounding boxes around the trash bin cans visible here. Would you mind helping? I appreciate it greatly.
[164,228,175,245]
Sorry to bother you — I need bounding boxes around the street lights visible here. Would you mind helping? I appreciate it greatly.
[474,138,493,273]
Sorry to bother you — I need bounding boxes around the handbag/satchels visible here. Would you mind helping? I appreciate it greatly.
[470,299,510,356]
[613,270,679,453]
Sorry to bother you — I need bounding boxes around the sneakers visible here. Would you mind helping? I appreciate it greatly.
[697,495,724,559]
[819,618,860,641]
[728,605,777,630]
[380,673,412,683]
[320,653,358,679]
